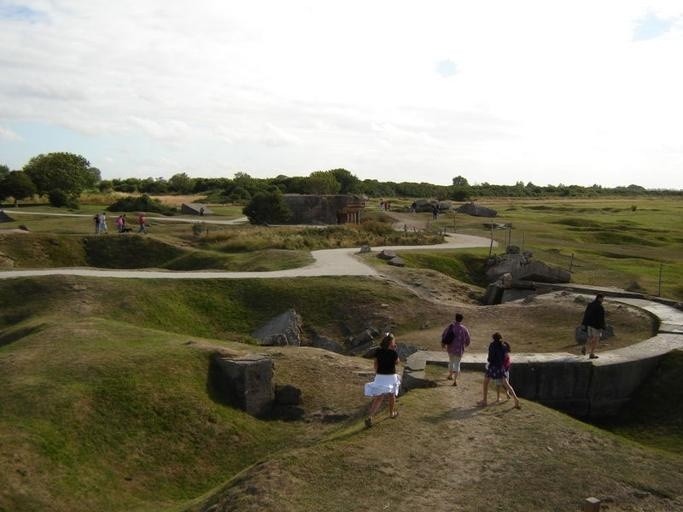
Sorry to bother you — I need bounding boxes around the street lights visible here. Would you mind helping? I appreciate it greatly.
[443,330,453,344]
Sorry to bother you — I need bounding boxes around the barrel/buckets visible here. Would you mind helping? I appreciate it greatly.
[390,411,399,418]
[365,418,372,428]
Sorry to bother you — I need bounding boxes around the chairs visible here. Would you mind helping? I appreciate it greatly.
[476,400,487,406]
[448,374,457,386]
[496,395,519,407]
[581,347,599,359]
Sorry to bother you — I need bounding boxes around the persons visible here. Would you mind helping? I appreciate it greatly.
[379,198,391,211]
[431,203,440,220]
[362,331,402,427]
[582,293,606,359]
[440,312,470,387]
[93,214,100,234]
[116,215,123,233]
[121,213,126,232]
[475,331,520,408]
[98,212,108,234]
[496,341,512,401]
[411,200,416,217]
[199,207,204,216]
[137,212,146,235]
